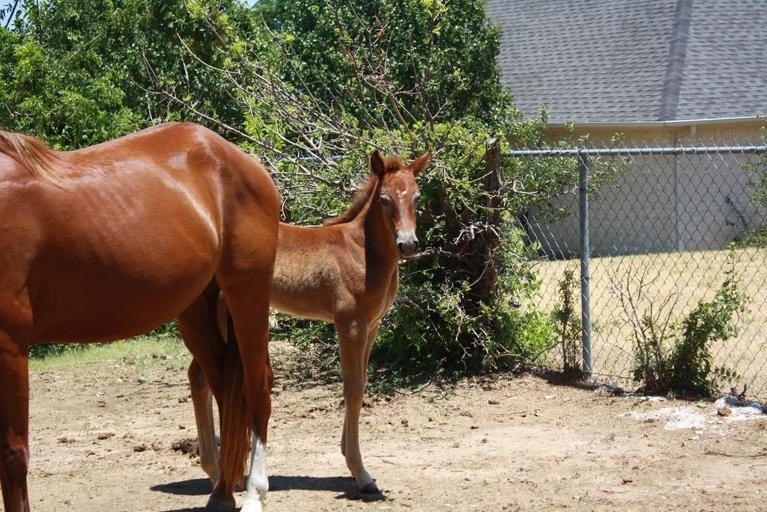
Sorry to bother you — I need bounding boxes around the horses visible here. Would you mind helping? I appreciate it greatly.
[186,149,435,501]
[0,121,284,511]
